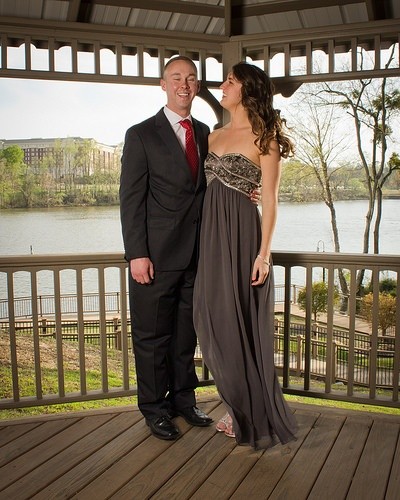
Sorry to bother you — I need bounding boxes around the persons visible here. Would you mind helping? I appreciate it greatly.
[120,56,261,442]
[192,62,300,451]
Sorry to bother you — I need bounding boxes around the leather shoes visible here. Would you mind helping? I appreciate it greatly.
[146,416,181,440]
[168,406,214,425]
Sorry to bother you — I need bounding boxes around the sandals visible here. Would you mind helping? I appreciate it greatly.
[225,426,239,437]
[216,412,233,431]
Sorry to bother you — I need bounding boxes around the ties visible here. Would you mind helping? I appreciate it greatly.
[179,120,198,186]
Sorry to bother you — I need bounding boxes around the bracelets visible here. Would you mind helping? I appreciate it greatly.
[258,255,271,266]
